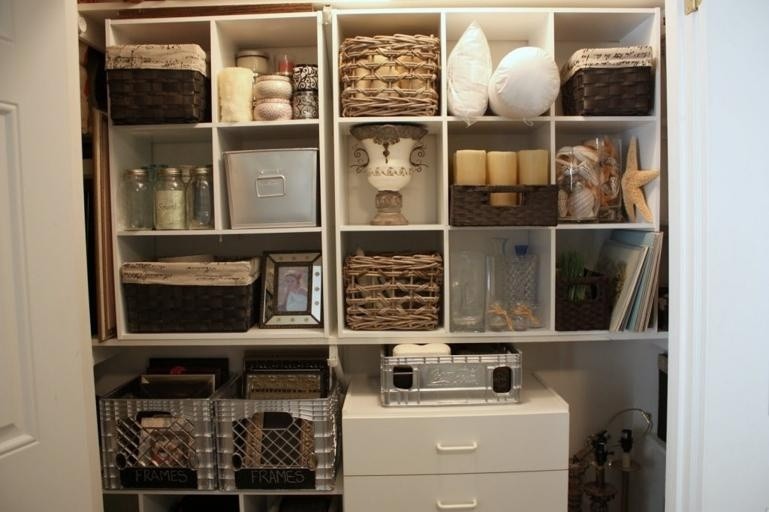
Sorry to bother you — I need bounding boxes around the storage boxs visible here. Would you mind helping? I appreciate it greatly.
[380,344,521,404]
[221,146,318,230]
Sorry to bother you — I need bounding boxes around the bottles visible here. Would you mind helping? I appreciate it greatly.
[154,169,188,229]
[189,168,213,230]
[119,170,153,230]
[504,243,537,308]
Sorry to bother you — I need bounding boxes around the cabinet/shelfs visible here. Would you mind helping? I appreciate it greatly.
[102,14,332,341]
[91,337,342,510]
[340,371,571,512]
[331,7,670,347]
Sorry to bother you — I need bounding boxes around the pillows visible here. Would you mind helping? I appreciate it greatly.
[488,45,562,128]
[447,19,494,128]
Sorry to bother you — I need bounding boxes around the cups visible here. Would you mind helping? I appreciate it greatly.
[450,250,490,332]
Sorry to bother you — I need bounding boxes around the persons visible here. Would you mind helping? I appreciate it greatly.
[285,272,307,312]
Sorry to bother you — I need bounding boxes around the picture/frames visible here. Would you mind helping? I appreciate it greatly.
[258,249,322,329]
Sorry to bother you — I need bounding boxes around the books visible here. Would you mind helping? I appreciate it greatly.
[594,229,664,332]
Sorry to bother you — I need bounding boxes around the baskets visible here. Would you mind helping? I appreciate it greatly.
[99,370,238,492]
[449,184,560,227]
[555,261,612,331]
[104,43,211,126]
[559,45,655,116]
[338,33,442,116]
[344,253,446,331]
[213,368,342,492]
[121,257,261,335]
